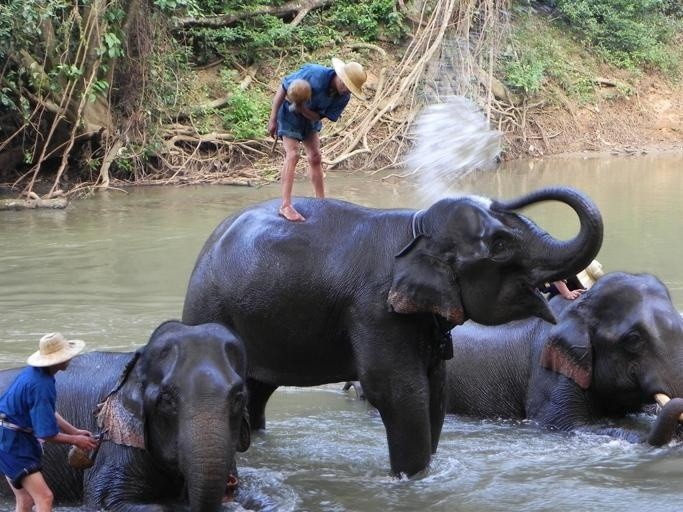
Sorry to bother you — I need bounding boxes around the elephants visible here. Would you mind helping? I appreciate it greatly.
[336,267,682,449]
[0,318,258,512]
[179,183,608,486]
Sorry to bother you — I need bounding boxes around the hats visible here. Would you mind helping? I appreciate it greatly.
[575,259,603,291]
[331,57,367,102]
[26,332,84,368]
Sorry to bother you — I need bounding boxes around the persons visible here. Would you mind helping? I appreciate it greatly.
[0,332,99,512]
[268,56,367,221]
[541,259,604,300]
